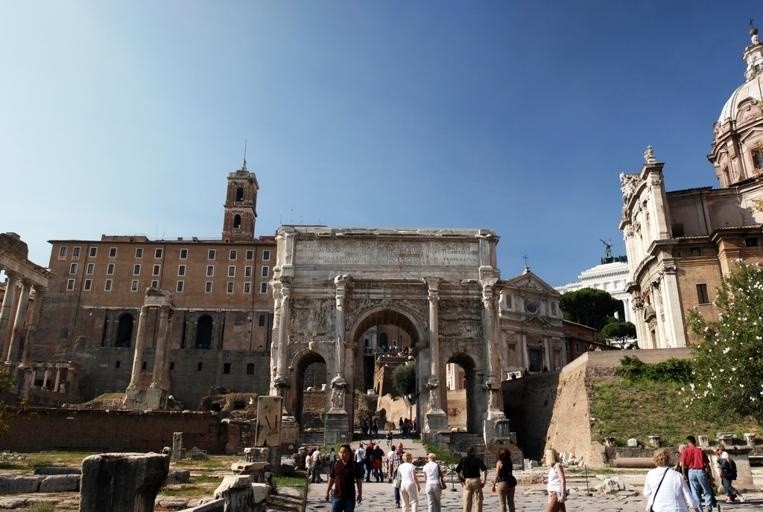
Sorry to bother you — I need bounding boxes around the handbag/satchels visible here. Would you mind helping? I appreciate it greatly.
[437,465,446,490]
[507,476,516,488]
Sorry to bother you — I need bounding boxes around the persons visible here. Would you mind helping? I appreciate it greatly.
[679,434,714,511]
[716,444,747,504]
[698,451,718,507]
[421,453,443,512]
[490,447,517,512]
[396,451,422,512]
[324,443,364,511]
[453,444,489,511]
[387,441,406,507]
[673,442,689,491]
[715,448,737,500]
[642,446,697,512]
[541,447,567,512]
[289,406,420,484]
